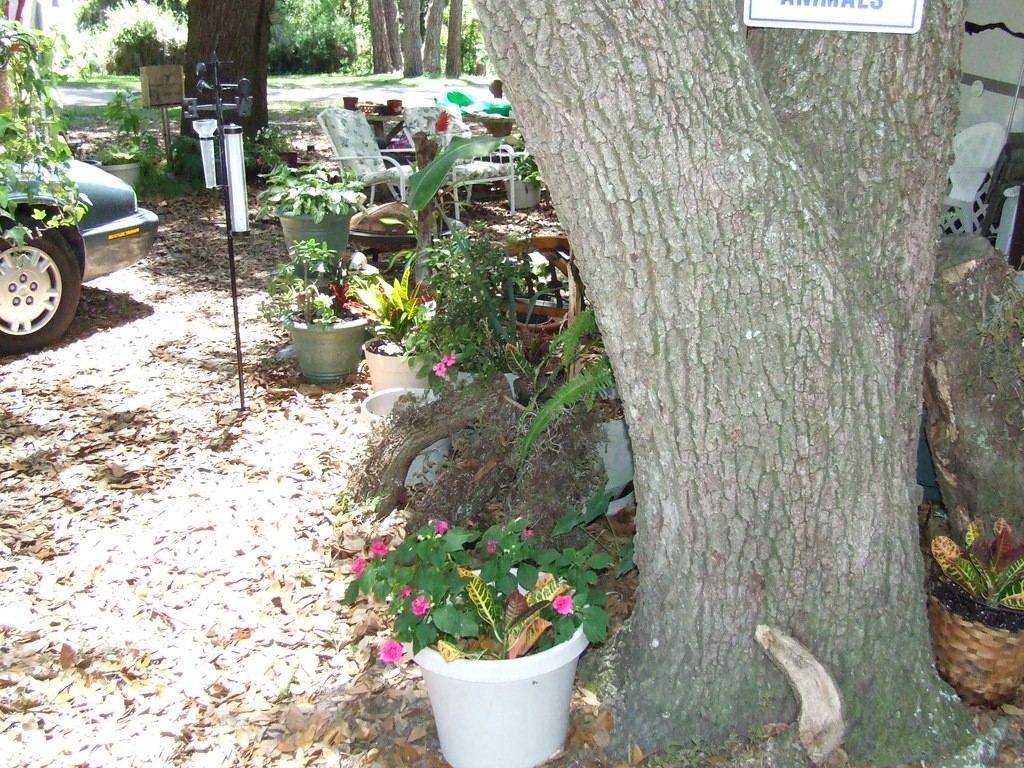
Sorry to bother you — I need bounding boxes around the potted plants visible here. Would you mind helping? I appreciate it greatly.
[98,144,159,189]
[504,154,543,209]
[930,518,1024,633]
[253,161,368,265]
[260,236,383,385]
[350,267,435,391]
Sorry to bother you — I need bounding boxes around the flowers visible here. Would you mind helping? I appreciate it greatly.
[400,222,532,396]
[254,126,290,154]
[345,517,609,659]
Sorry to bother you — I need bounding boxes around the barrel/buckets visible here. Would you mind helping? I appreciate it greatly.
[360,388,449,489]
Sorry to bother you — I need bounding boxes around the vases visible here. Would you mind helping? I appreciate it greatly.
[342,97,359,110]
[387,99,402,115]
[404,567,592,768]
[448,369,522,401]
[278,152,297,168]
[361,387,452,486]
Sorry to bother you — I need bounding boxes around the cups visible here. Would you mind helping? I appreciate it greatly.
[387,100,401,115]
[343,97,358,111]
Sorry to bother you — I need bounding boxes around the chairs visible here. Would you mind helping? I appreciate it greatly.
[401,106,514,225]
[942,121,1007,235]
[995,186,1024,293]
[318,108,462,240]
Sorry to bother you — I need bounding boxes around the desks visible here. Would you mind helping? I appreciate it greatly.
[463,111,516,138]
[366,114,406,139]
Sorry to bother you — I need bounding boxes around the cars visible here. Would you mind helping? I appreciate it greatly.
[0,158,160,351]
